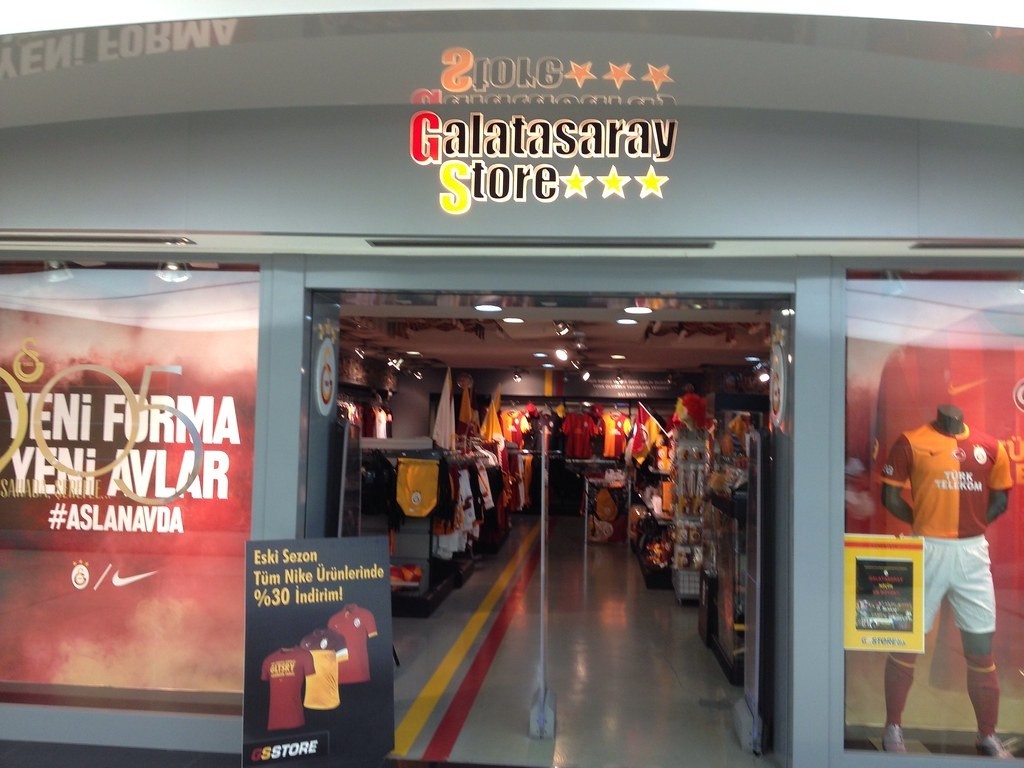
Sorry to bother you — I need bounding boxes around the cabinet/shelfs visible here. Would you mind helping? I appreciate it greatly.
[671,442,705,608]
[711,493,747,686]
[360,436,453,618]
[695,392,769,649]
[635,473,675,590]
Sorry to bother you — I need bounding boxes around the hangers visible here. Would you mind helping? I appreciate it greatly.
[366,447,478,464]
[576,402,584,415]
[542,402,552,416]
[338,391,353,403]
[611,402,621,416]
[509,401,520,414]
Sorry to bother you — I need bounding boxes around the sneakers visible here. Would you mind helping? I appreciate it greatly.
[882,725,905,752]
[975,734,1015,759]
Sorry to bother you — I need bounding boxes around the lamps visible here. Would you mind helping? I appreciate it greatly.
[571,355,589,370]
[553,320,570,337]
[758,366,770,383]
[555,340,578,362]
[578,369,591,383]
[512,366,522,383]
[413,367,424,380]
[354,340,368,360]
[387,352,404,371]
[616,369,623,382]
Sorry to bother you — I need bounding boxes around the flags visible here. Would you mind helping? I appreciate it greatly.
[431,366,455,453]
[480,379,503,440]
[459,380,472,426]
[632,400,662,466]
[543,368,564,397]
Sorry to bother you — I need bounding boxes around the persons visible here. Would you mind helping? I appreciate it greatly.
[879,402,1015,759]
